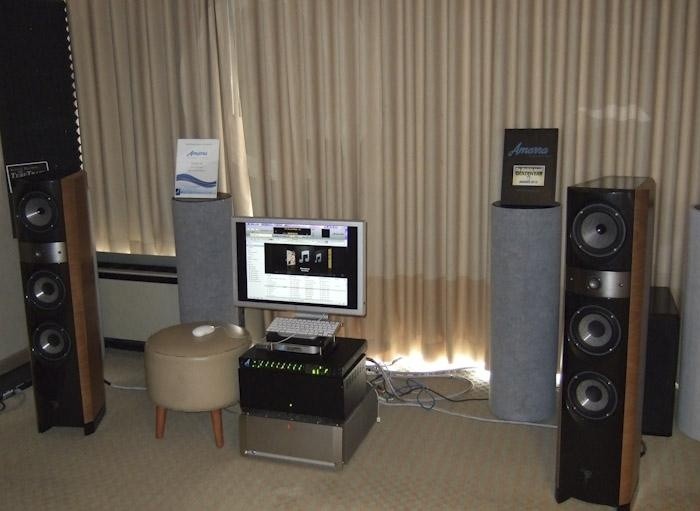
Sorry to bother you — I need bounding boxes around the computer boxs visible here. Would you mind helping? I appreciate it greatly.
[238,352,368,420]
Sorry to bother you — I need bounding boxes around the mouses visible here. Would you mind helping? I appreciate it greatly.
[192,325,216,337]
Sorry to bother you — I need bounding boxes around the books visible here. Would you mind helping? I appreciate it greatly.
[174,139,220,199]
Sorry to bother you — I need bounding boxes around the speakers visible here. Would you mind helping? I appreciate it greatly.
[11,169,107,436]
[641,285,680,437]
[0,0,84,195]
[554,174,658,511]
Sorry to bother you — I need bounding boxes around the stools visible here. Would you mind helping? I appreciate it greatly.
[142,320,253,448]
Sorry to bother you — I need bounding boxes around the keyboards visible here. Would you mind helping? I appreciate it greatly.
[265,316,343,338]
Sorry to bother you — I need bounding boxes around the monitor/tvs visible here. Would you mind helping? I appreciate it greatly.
[230,214,368,319]
